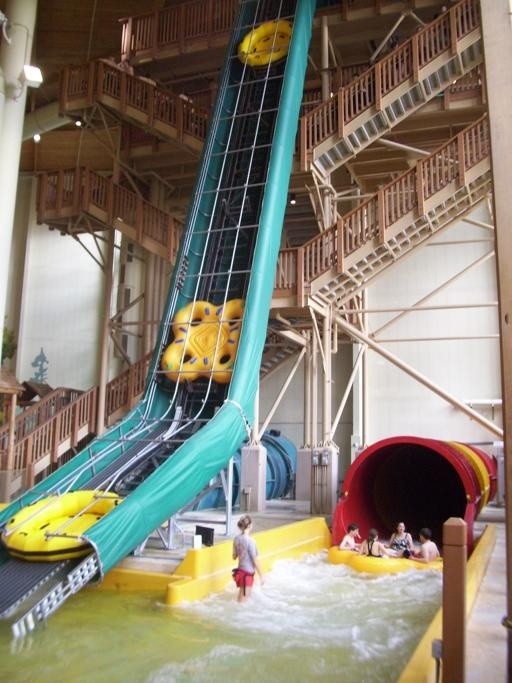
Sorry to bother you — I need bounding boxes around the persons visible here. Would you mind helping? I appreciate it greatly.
[357,528,399,558]
[337,523,362,552]
[396,527,441,564]
[72,55,208,137]
[230,515,267,604]
[383,521,414,554]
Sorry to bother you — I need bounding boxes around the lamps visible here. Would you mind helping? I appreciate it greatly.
[1,13,42,102]
